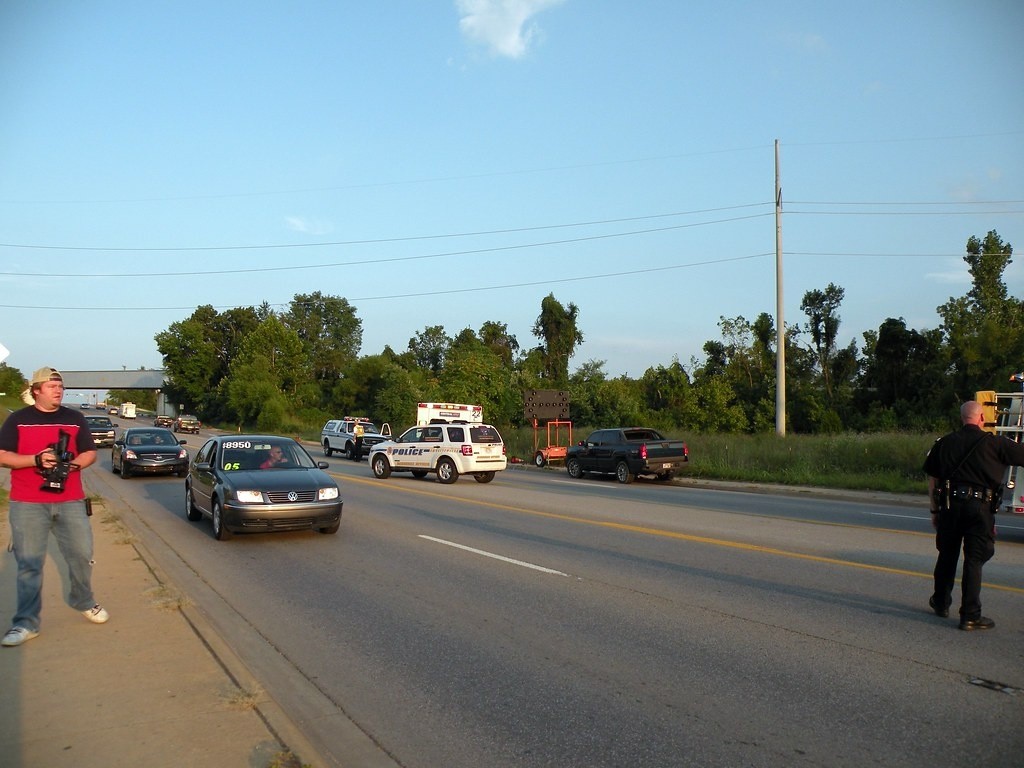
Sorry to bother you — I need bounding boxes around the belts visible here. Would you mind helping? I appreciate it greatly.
[943,488,992,502]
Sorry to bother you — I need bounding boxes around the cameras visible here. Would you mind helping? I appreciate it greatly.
[39,436,81,493]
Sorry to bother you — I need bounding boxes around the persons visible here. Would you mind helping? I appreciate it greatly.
[353,421,364,462]
[259,446,287,469]
[0,367,108,645]
[923,401,1024,632]
[132,436,142,444]
[152,434,164,446]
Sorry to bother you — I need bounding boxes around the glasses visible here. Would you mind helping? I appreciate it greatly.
[274,451,283,453]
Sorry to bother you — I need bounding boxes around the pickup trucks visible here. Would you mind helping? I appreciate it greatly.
[565,426,688,485]
[174,414,201,434]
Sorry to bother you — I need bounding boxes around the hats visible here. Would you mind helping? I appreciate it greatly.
[30,366,63,386]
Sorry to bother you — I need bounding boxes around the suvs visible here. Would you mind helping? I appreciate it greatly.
[368,418,507,484]
[320,416,392,460]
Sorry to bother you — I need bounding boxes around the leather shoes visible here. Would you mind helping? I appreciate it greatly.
[929,595,950,618]
[960,616,996,630]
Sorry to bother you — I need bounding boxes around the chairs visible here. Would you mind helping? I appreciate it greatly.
[431,432,438,437]
[129,436,142,444]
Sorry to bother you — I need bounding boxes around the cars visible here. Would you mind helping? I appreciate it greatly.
[79,403,118,414]
[153,415,173,428]
[85,416,119,448]
[112,427,190,479]
[185,435,343,540]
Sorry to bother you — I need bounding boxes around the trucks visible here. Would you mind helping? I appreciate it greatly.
[121,403,137,418]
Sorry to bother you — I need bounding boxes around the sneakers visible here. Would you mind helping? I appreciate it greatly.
[0,627,39,645]
[80,604,110,624]
